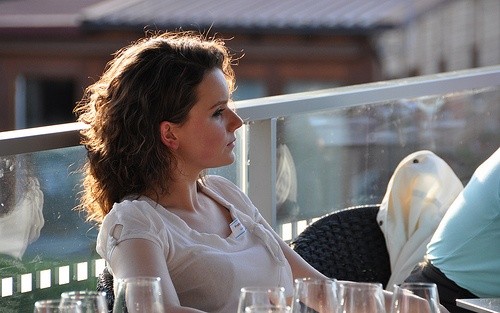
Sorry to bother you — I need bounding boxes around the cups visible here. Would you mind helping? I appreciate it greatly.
[58,290,109,313]
[35,299,82,313]
[112,276,164,313]
[237,277,442,313]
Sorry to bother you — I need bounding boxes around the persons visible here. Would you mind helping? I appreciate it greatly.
[73,32,448,313]
[400,147,500,313]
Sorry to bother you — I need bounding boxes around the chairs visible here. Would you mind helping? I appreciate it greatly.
[292,202,392,291]
